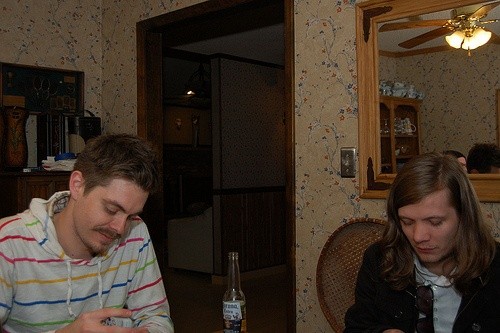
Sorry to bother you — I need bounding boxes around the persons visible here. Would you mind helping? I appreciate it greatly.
[466,143,500,174]
[440,150,466,173]
[344,154,500,333]
[0,133,175,333]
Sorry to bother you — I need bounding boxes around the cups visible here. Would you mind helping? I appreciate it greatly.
[380,118,416,135]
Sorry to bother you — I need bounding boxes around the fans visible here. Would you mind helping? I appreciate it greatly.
[379,2,500,49]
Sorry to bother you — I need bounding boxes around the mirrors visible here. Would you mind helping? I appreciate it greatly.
[356,0,500,203]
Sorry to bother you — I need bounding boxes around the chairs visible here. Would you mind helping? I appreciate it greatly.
[168,207,213,287]
[316,219,388,333]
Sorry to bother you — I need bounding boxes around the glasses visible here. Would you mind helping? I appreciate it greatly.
[416,285,435,333]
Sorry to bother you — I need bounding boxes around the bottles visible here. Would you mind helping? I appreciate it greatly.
[379,80,418,98]
[222,252,248,333]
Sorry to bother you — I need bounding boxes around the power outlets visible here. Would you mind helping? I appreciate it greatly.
[340,148,355,177]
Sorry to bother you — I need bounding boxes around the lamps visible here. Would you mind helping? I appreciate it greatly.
[445,14,493,56]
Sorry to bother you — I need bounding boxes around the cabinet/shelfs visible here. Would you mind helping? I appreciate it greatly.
[0,62,85,168]
[0,172,70,219]
[377,94,419,178]
